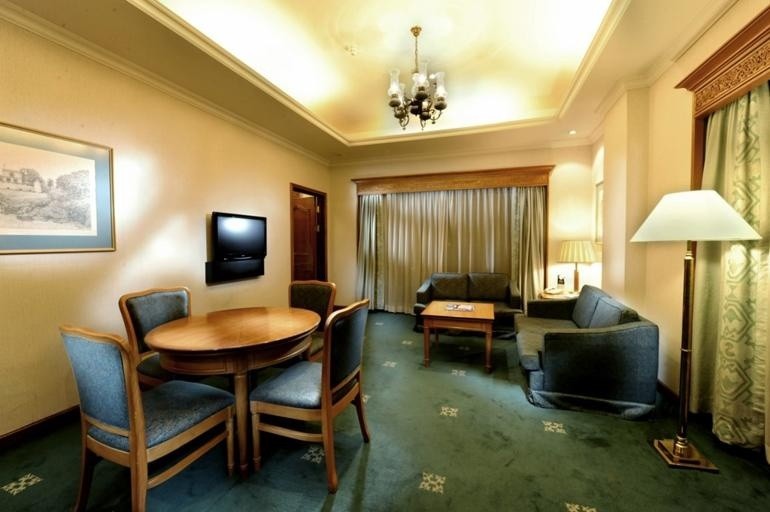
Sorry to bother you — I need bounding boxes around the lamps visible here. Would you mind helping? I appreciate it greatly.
[557,240,598,291]
[627,189,767,474]
[388,21,449,133]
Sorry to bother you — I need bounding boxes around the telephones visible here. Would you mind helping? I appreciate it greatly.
[544,287,562,295]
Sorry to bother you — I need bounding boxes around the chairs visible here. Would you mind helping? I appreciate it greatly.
[58,322,239,511]
[118,285,236,457]
[275,277,338,369]
[248,298,377,494]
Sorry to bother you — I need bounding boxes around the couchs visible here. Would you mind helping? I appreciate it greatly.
[412,270,521,340]
[514,283,660,423]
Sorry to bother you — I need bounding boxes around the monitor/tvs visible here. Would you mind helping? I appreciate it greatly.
[212,212,267,262]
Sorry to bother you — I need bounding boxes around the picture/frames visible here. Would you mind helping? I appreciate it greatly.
[594,179,605,246]
[0,122,116,262]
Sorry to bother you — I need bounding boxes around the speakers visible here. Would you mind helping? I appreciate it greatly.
[206,259,264,284]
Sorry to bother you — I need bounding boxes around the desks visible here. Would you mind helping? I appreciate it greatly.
[419,300,498,374]
[538,287,584,300]
[141,305,322,475]
